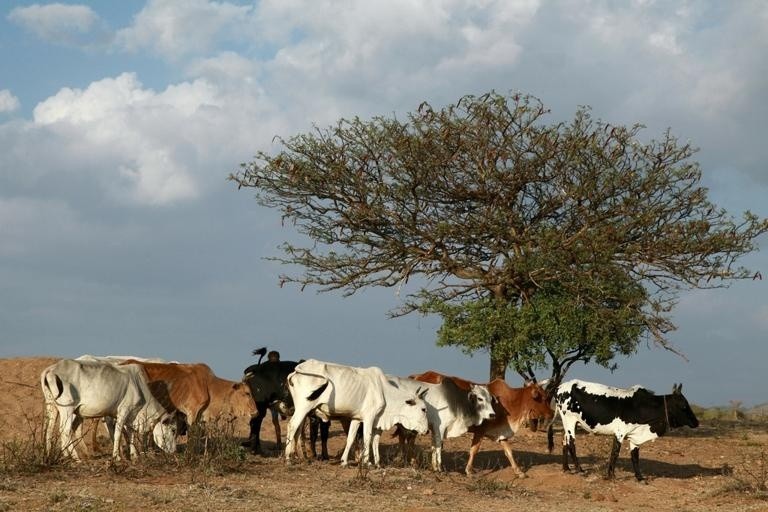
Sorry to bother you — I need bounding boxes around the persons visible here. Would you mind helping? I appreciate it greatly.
[266,349,288,451]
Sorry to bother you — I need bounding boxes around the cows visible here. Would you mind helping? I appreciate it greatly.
[42,347,257,471]
[247,348,556,481]
[547,378,700,485]
